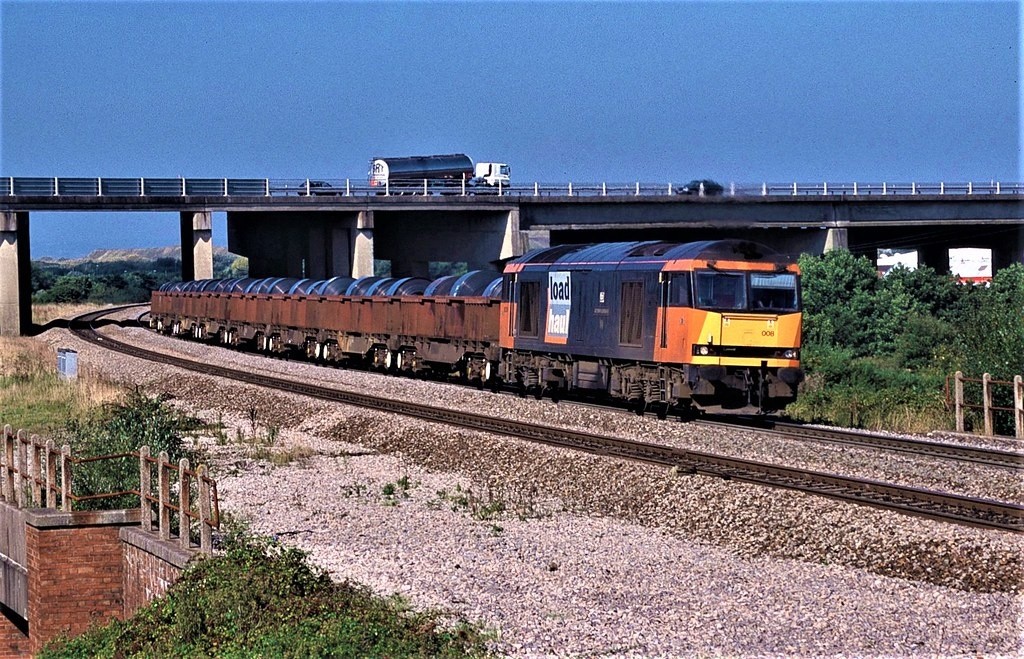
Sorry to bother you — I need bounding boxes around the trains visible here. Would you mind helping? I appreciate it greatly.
[149,238,803,422]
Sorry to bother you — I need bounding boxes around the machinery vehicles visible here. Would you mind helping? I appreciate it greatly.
[369,154,511,196]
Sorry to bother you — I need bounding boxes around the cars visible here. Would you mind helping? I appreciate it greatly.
[298,180,344,197]
[675,180,724,197]
